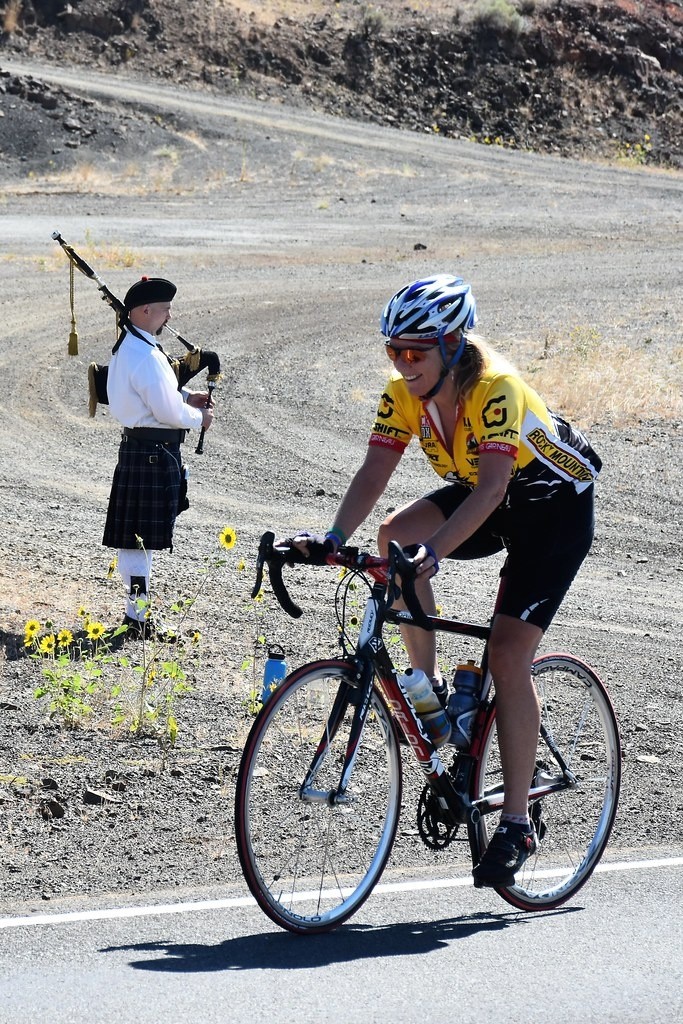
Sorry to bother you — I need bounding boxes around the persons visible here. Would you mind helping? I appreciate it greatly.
[102,277,215,643]
[275,274,602,880]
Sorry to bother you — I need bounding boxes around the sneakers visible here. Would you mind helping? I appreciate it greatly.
[471,818,539,883]
[392,677,450,743]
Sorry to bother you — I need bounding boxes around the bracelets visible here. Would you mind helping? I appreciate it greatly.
[326,533,342,548]
[326,527,347,544]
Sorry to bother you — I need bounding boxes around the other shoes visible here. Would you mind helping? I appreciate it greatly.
[121,612,174,643]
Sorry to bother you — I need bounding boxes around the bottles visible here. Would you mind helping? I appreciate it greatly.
[446,659,483,751]
[261,643,286,705]
[401,667,452,748]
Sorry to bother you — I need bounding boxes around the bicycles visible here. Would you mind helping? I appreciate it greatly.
[232,524,621,934]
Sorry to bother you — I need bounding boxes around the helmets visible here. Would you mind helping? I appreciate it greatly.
[379,274,478,340]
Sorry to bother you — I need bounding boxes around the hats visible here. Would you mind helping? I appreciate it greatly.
[123,275,177,310]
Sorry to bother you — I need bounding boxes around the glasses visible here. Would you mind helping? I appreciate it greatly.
[383,341,436,364]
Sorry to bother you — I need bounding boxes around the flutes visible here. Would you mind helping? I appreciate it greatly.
[52,231,220,455]
